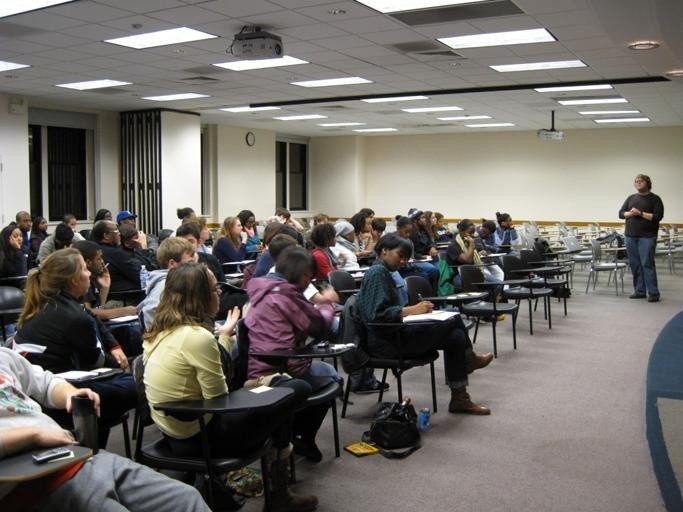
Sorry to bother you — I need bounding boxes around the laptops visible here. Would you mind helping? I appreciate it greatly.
[556,236,581,253]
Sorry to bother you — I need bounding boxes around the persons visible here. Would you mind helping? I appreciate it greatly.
[619,174,664,302]
[2,207,338,512]
[332,207,520,415]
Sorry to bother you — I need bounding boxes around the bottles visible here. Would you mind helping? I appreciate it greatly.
[401,396,411,407]
[139,265,149,290]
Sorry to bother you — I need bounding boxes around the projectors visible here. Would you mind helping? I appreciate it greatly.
[233,37,283,58]
[540,130,564,140]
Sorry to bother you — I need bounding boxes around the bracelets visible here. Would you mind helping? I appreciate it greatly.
[639,211,643,217]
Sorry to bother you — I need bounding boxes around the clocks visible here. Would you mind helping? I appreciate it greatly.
[246,132,255,146]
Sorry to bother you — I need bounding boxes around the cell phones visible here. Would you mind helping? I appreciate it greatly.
[318,341,329,348]
[32,447,70,464]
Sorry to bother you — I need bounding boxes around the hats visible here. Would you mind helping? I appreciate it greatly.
[335,221,354,235]
[116,211,137,221]
[55,223,75,239]
[408,207,422,221]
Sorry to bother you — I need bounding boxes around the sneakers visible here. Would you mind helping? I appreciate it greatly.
[352,379,390,394]
[265,489,320,512]
[465,350,494,377]
[448,390,490,417]
[627,291,660,303]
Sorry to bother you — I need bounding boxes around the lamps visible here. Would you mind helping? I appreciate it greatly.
[8,96,26,114]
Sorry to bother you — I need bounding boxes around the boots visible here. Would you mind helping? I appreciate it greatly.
[272,446,305,475]
